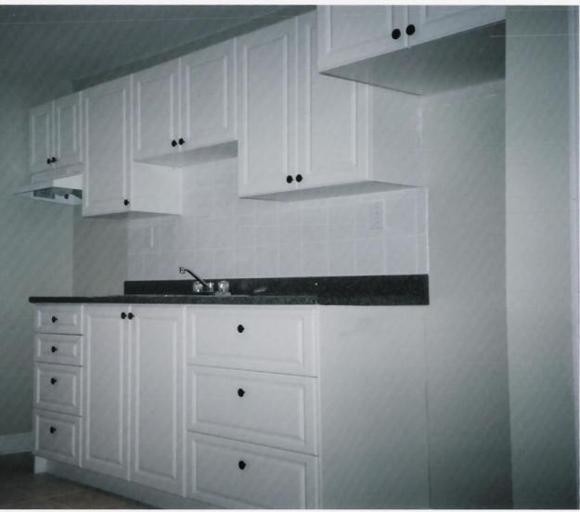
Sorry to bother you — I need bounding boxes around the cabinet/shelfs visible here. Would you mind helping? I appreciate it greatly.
[84,77,180,220]
[238,7,425,205]
[136,33,238,165]
[27,93,84,173]
[184,300,433,508]
[86,304,183,508]
[30,299,86,488]
[315,4,506,96]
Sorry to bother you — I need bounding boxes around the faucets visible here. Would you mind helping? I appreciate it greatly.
[179,266,216,293]
